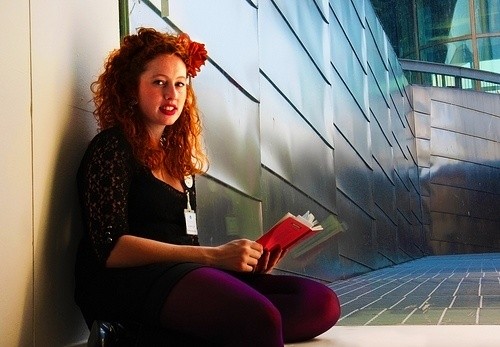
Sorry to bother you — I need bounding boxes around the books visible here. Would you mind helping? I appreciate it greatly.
[252,209,326,254]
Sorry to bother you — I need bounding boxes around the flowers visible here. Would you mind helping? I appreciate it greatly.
[185,42,208,78]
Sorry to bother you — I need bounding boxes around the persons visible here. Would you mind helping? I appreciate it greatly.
[69,27,341,347]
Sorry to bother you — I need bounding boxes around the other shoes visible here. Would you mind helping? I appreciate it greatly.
[87,320,119,347]
